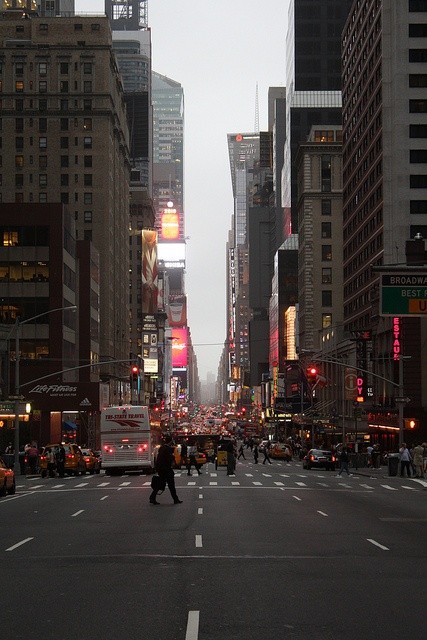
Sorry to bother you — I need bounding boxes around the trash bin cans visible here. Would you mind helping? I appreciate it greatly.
[385,455,398,476]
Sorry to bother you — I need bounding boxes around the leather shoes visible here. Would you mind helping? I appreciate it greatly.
[174,500,183,505]
[149,499,160,505]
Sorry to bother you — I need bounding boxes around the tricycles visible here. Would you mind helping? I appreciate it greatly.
[215,445,236,471]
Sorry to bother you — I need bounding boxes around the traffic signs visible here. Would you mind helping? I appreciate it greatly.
[381,272,427,318]
[6,305,79,395]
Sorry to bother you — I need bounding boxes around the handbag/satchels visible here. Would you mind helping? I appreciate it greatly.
[151,476,166,490]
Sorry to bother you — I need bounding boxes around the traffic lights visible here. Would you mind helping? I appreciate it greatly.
[132,365,139,373]
[310,366,316,375]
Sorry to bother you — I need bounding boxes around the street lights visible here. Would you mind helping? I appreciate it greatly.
[299,347,347,440]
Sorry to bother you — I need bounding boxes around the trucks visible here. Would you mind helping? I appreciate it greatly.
[99,405,158,476]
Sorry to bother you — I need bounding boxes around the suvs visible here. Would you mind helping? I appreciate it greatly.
[39,443,85,475]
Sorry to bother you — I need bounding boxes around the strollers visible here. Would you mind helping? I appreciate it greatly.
[0,452,15,497]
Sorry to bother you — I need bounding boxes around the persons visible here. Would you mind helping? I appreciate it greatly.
[29,444,39,474]
[337,445,354,478]
[421,443,426,472]
[179,439,191,469]
[262,445,273,465]
[149,435,183,505]
[57,443,67,478]
[408,443,416,476]
[53,446,61,478]
[187,440,203,477]
[284,445,293,465]
[47,448,56,478]
[293,442,297,454]
[359,443,382,470]
[23,445,32,474]
[296,443,301,455]
[399,443,412,476]
[412,441,424,478]
[237,444,245,460]
[253,446,260,465]
[226,439,236,478]
[5,441,12,453]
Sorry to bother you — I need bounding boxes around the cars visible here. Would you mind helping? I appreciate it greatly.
[303,447,337,471]
[82,447,98,475]
[259,440,293,460]
[176,445,207,469]
